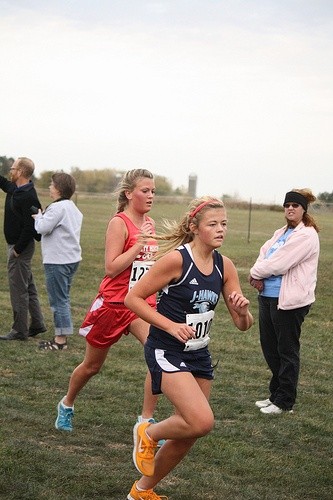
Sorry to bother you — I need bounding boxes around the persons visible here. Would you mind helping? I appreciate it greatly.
[124,197,254,500]
[54,168,170,449]
[0,156,49,341]
[247,188,319,414]
[31,172,83,354]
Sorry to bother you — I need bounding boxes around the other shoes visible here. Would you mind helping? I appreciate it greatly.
[36,342,68,354]
[26,324,47,337]
[0,329,29,341]
[38,337,55,349]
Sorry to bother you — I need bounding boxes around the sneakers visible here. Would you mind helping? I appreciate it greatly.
[55,398,75,433]
[131,421,159,477]
[255,398,273,408]
[126,479,168,500]
[260,403,294,415]
[133,416,164,444]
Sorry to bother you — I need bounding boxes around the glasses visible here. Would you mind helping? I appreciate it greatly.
[283,203,301,208]
